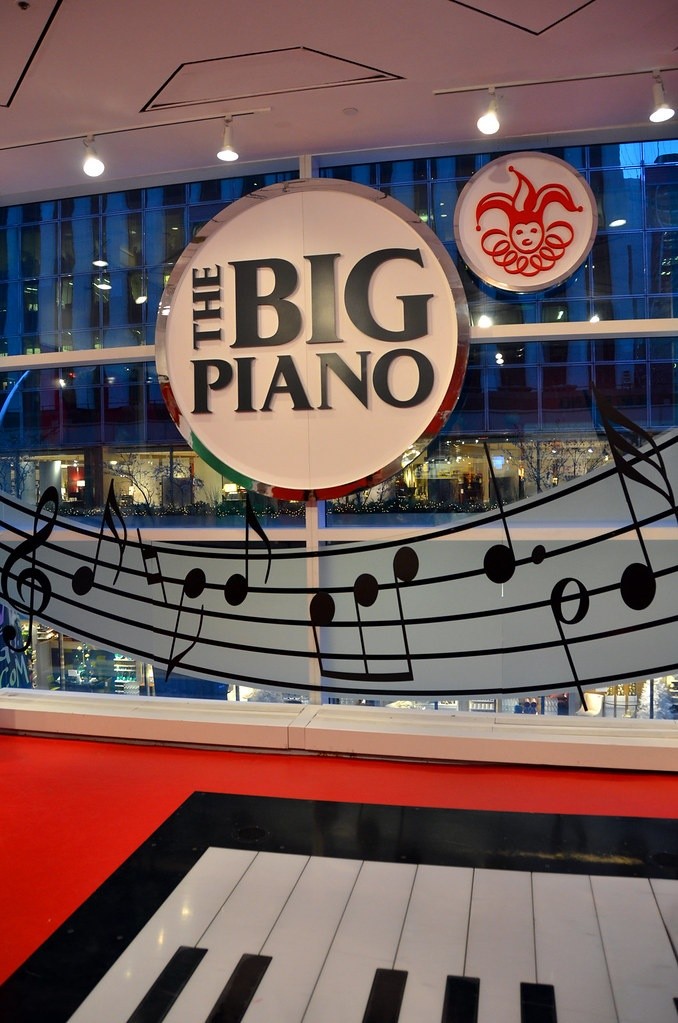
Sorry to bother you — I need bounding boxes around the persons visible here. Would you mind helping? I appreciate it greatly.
[396,479,407,487]
[77,488,83,500]
[462,477,469,492]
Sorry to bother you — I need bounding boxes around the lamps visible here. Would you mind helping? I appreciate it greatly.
[649,81,675,123]
[603,192,627,227]
[83,133,105,177]
[475,96,500,135]
[91,232,112,291]
[216,120,239,162]
[129,247,183,305]
